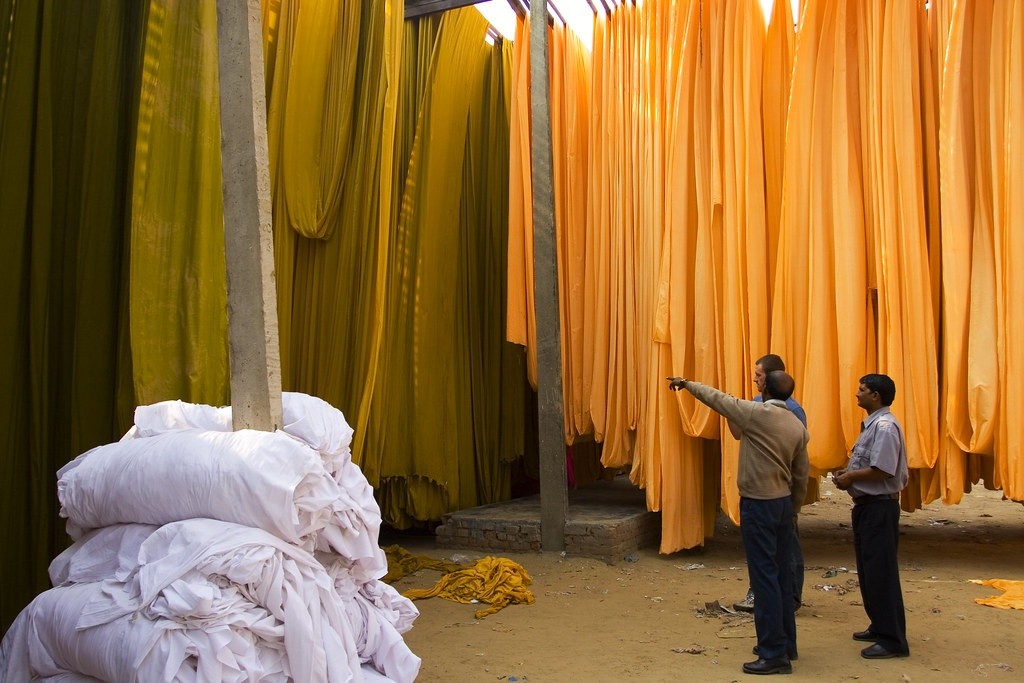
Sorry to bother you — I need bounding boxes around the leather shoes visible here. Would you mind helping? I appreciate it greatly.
[853,629,877,643]
[742,657,792,675]
[753,645,798,661]
[861,643,899,659]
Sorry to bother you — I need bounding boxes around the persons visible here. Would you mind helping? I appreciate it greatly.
[833,374,911,659]
[726,354,807,613]
[665,371,809,675]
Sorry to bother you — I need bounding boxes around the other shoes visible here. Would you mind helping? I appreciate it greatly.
[741,595,754,604]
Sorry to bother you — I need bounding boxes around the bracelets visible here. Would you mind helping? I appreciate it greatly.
[680,378,688,387]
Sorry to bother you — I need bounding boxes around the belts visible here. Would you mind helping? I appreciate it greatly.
[852,492,899,505]
